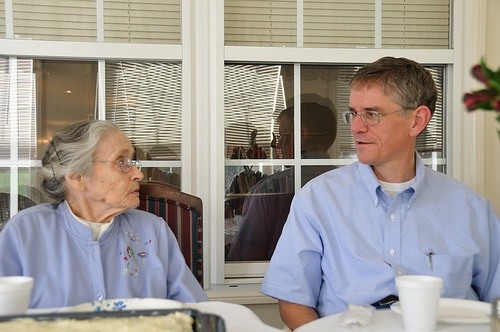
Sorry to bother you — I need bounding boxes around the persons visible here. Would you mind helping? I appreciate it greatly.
[225,92,338,262]
[0,119,209,309]
[257,56,500,332]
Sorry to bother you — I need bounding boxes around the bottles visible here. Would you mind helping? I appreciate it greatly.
[490,299,500,332]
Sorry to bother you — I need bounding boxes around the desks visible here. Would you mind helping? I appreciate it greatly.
[293,307,500,332]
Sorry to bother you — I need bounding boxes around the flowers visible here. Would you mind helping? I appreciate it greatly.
[463,58,500,138]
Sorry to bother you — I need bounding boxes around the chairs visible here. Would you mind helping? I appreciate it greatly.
[136,184,204,289]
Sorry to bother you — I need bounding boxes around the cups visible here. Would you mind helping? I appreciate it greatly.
[0,276,34,316]
[394,275,443,332]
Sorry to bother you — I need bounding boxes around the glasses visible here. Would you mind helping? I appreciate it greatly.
[94,157,143,173]
[343,109,403,125]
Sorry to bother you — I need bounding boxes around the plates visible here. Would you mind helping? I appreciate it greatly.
[70,298,182,312]
[390,298,493,324]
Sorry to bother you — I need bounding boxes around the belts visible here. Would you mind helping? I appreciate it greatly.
[370,294,398,310]
